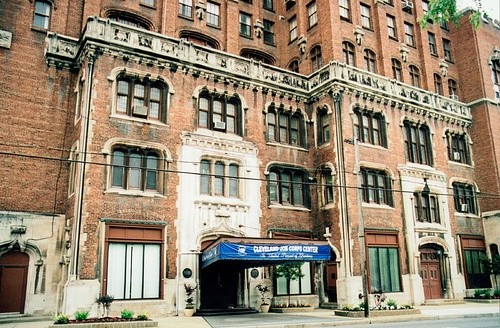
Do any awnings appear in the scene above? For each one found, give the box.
[198,237,329,268]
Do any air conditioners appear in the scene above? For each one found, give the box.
[461,204,467,212]
[133,105,148,116]
[214,121,226,129]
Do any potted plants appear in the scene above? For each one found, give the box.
[184,284,197,316]
[256,285,271,313]
